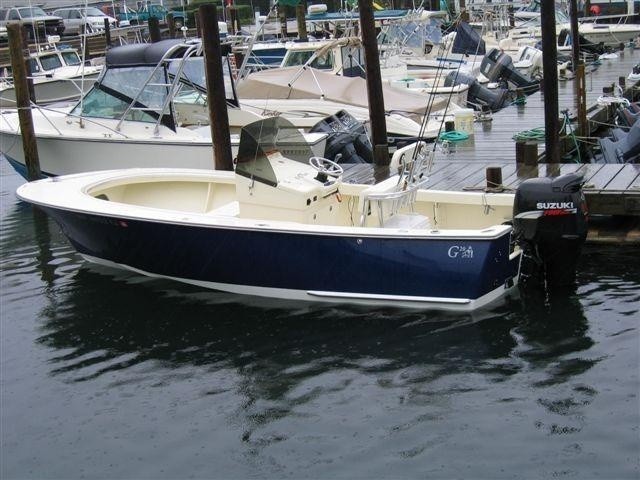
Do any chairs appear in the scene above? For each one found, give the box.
[356,139,427,219]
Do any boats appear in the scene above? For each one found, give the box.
[26,277,585,374]
[13,116,595,325]
[0,0,640,184]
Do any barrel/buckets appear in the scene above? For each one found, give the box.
[455,108,474,134]
